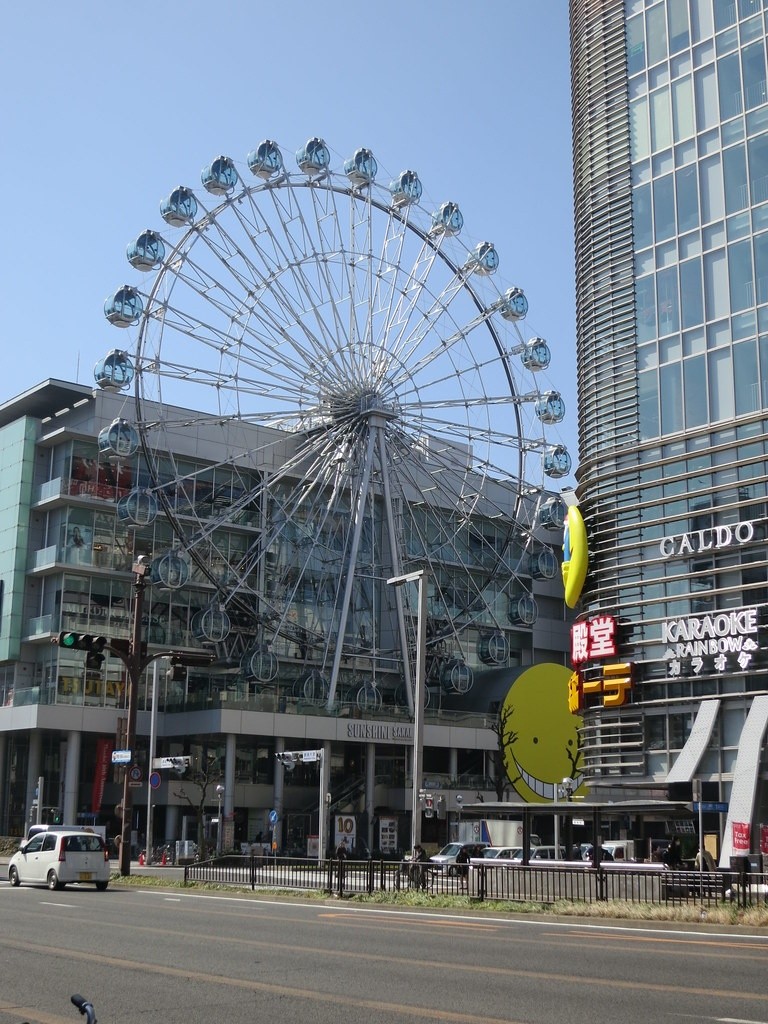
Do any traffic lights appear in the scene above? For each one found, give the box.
[58,631,106,653]
[169,649,211,668]
[165,667,187,680]
[275,752,293,763]
[424,798,434,818]
[437,800,447,820]
[83,651,106,669]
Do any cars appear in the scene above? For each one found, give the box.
[481,839,635,867]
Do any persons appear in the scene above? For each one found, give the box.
[36,836,53,850]
[472,845,484,858]
[585,841,614,860]
[412,845,426,888]
[695,846,717,895]
[256,831,263,842]
[652,835,683,870]
[337,841,347,877]
[68,837,79,850]
[456,848,470,889]
[573,843,582,860]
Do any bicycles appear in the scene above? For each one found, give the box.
[392,859,435,889]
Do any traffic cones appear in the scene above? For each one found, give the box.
[139,853,144,865]
[161,853,167,866]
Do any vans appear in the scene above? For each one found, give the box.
[27,826,85,842]
[428,842,492,877]
[8,831,111,891]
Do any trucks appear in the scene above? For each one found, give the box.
[450,819,542,848]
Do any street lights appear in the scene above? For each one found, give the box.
[386,569,432,862]
